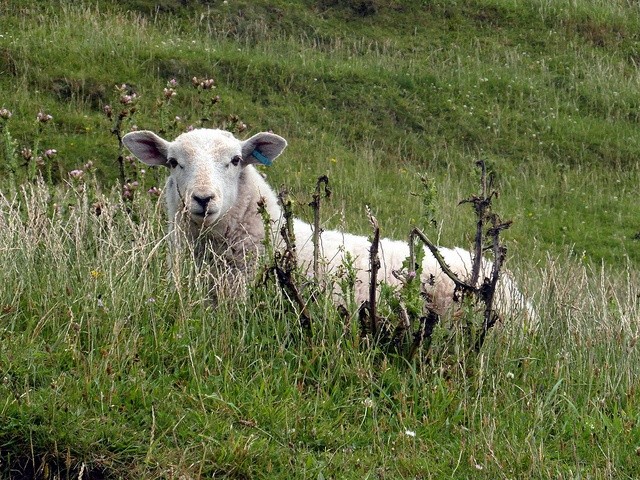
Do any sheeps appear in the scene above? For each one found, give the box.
[121,127,536,334]
